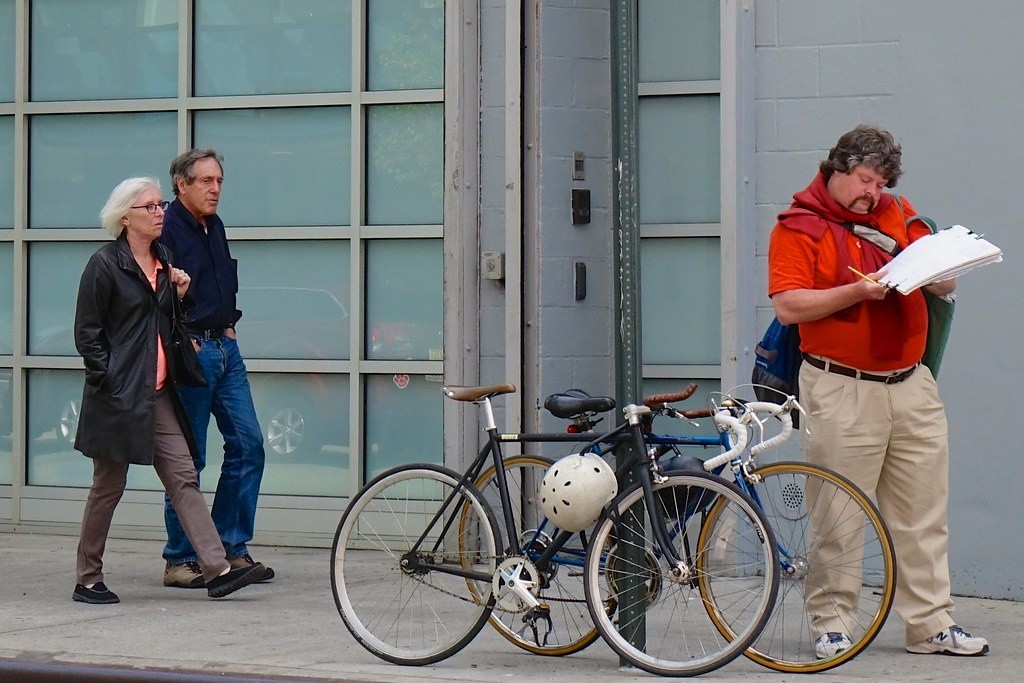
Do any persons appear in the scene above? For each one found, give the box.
[767,124,990,660]
[156,150,276,589]
[71,176,264,606]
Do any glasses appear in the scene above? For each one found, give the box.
[131,201,170,214]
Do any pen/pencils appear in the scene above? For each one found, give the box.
[848,265,892,293]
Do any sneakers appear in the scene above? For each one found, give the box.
[904,625,990,656]
[815,633,852,659]
[164,561,208,588]
[226,554,276,583]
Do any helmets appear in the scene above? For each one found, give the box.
[539,452,618,533]
[650,455,718,520]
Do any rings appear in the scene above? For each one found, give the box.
[184,273,188,276]
[181,270,184,274]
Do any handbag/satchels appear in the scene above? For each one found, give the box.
[159,243,209,389]
[752,316,804,430]
[894,194,958,382]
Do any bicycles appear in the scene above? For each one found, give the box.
[458,388,900,672]
[326,377,780,677]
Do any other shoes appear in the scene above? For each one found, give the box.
[73,581,121,604]
[206,562,266,598]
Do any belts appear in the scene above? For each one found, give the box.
[196,328,224,341]
[802,351,920,385]
